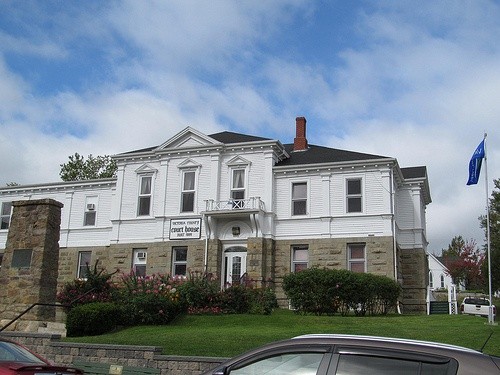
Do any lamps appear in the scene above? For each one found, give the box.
[232,227,240,235]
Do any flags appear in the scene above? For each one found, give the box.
[466,139,484,186]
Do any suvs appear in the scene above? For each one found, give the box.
[200,332,500,375]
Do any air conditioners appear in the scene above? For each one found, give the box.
[137,252,145,258]
[87,204,94,210]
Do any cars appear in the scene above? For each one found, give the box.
[0,335,85,375]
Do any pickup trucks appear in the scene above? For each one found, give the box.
[460,296,496,321]
